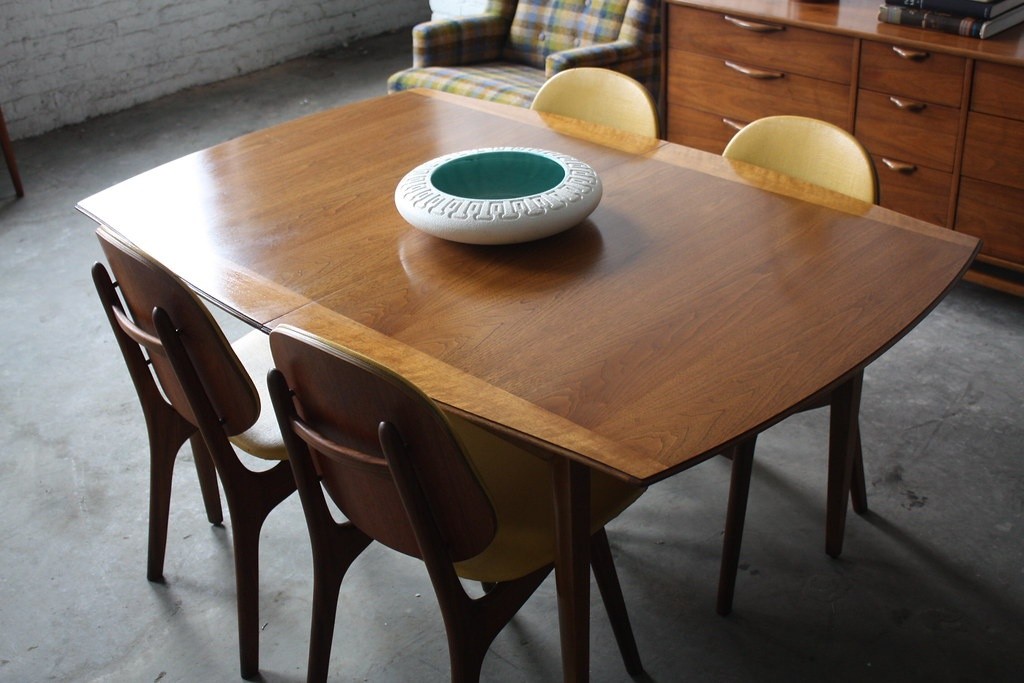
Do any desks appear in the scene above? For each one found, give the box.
[74,86,982,683]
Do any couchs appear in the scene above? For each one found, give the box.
[388,0,661,116]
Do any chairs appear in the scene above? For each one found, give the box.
[91,223,298,680]
[266,323,642,683]
[530,67,660,139]
[717,116,880,616]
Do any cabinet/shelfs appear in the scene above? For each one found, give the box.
[659,0,1024,297]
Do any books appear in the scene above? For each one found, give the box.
[886,0,1024,20]
[878,5,1024,40]
